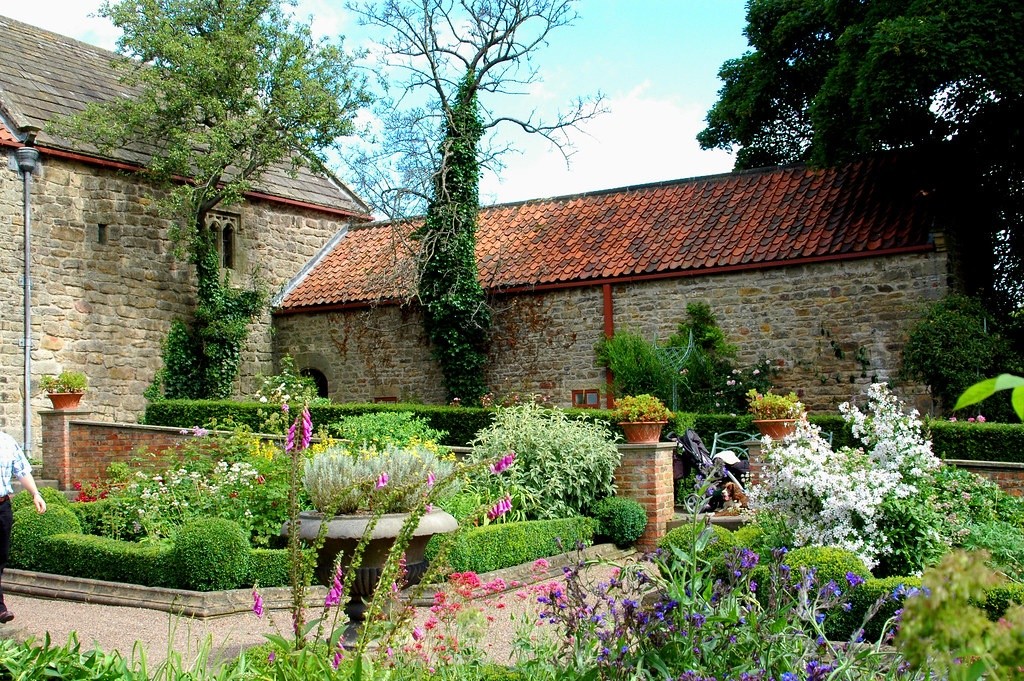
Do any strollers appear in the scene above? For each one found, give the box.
[666,428,749,513]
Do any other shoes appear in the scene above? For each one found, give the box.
[0,610,13,624]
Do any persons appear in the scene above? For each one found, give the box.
[0,430,47,624]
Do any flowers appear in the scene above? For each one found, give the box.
[611,392,676,422]
[746,385,808,420]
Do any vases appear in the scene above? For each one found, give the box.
[750,417,798,439]
[617,421,668,444]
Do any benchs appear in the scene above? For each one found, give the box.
[710,430,833,460]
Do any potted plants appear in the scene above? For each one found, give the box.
[280,439,459,659]
[38,372,88,410]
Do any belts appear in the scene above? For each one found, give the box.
[0,495,11,501]
